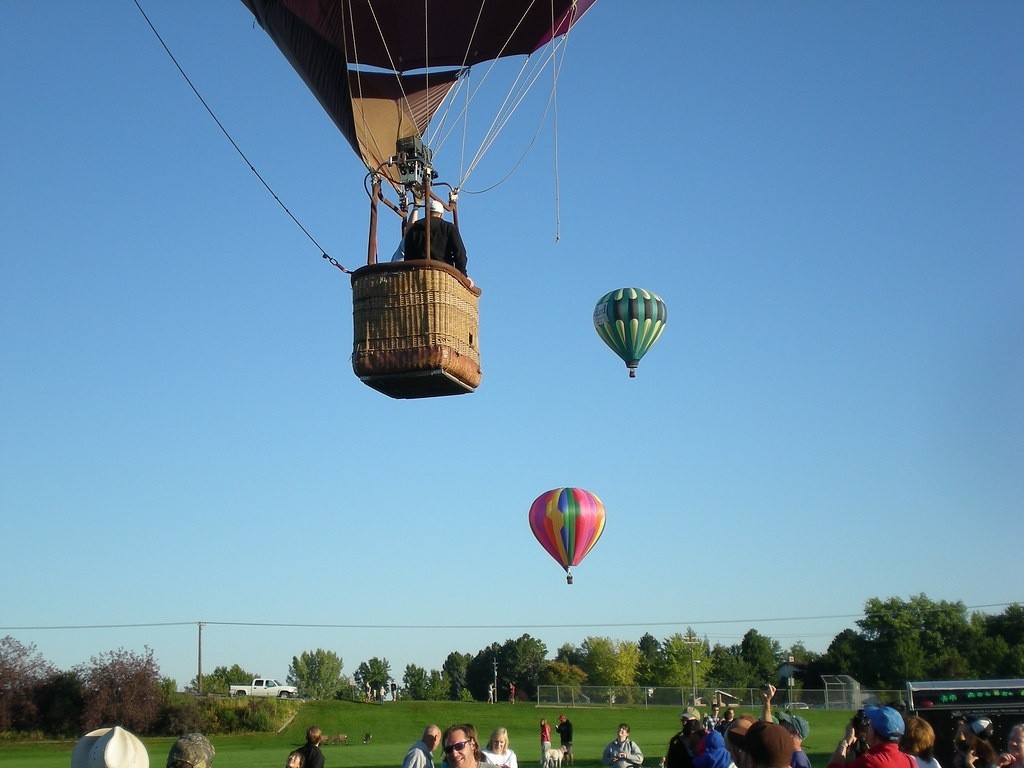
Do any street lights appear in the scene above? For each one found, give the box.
[692,660,701,706]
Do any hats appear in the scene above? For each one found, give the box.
[430,201,444,214]
[558,715,566,719]
[972,718,994,739]
[168,733,215,768]
[70,726,150,768]
[681,706,701,720]
[776,711,810,741]
[865,704,905,740]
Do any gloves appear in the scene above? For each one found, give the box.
[467,276,474,288]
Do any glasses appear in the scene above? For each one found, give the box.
[444,739,470,754]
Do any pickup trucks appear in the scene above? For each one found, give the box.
[228,678,299,698]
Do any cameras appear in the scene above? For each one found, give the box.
[853,709,870,727]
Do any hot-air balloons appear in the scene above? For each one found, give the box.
[528,487,607,584]
[593,286,668,379]
[135,1,599,399]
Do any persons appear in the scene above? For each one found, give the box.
[603,724,643,768]
[391,681,397,701]
[380,686,386,706]
[403,725,441,768]
[390,201,475,289]
[664,683,812,768]
[488,683,496,705]
[703,704,721,730]
[286,751,305,768]
[441,724,503,768]
[994,723,1024,768]
[555,715,574,766]
[540,719,551,765]
[290,726,325,768]
[507,682,515,704]
[714,708,736,737]
[166,732,215,768]
[826,701,1000,768]
[480,727,517,768]
[453,723,510,768]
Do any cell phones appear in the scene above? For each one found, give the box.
[760,684,769,691]
